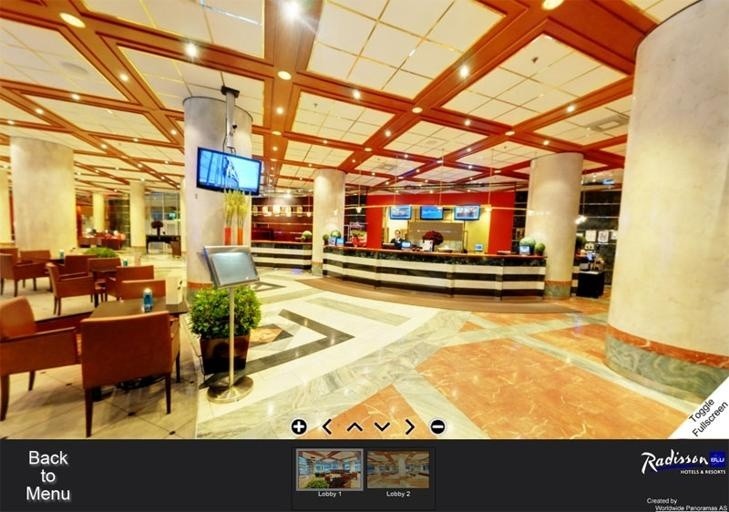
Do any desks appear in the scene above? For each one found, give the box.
[146,235,181,255]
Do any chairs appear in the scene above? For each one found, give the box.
[0,242,180,437]
[79,231,127,249]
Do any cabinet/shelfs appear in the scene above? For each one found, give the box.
[513,189,621,218]
[251,213,366,237]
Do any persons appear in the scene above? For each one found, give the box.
[391,229,404,250]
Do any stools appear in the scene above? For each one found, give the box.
[576,271,606,298]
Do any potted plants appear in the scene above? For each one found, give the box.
[535,243,545,256]
[301,231,313,242]
[323,235,329,245]
[151,221,163,237]
[222,188,247,245]
[188,287,262,374]
[331,230,341,245]
[519,237,536,255]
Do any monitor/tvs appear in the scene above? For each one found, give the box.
[519,245,530,256]
[196,146,262,196]
[454,206,480,221]
[420,205,443,220]
[402,242,411,248]
[474,242,484,252]
[388,206,412,220]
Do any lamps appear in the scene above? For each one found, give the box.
[355,148,363,213]
[306,191,312,218]
[484,135,494,212]
